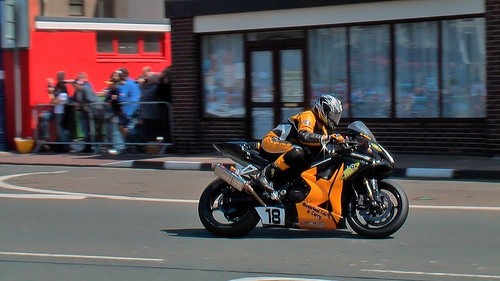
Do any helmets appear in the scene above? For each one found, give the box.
[312,94,343,130]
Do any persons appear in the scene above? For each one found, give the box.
[257,95,349,192]
[46,67,173,155]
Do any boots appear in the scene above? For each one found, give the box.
[253,161,284,192]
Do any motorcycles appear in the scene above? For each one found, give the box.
[197,121,410,239]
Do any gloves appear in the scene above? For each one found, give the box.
[321,133,345,144]
